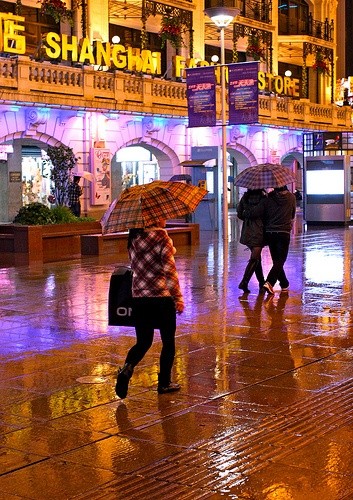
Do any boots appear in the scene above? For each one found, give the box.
[157,372,182,394]
[238,259,258,293]
[115,363,134,399]
[255,266,266,288]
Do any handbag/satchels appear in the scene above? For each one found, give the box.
[106,270,137,326]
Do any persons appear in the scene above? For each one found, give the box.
[293,188,302,200]
[236,189,269,293]
[116,220,185,398]
[68,176,83,217]
[242,186,297,294]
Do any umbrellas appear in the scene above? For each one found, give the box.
[233,162,298,191]
[99,180,209,235]
[70,170,96,184]
[169,174,193,180]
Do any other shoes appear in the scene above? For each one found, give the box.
[263,281,274,294]
[282,285,290,290]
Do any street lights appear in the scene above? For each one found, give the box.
[202,5,238,290]
